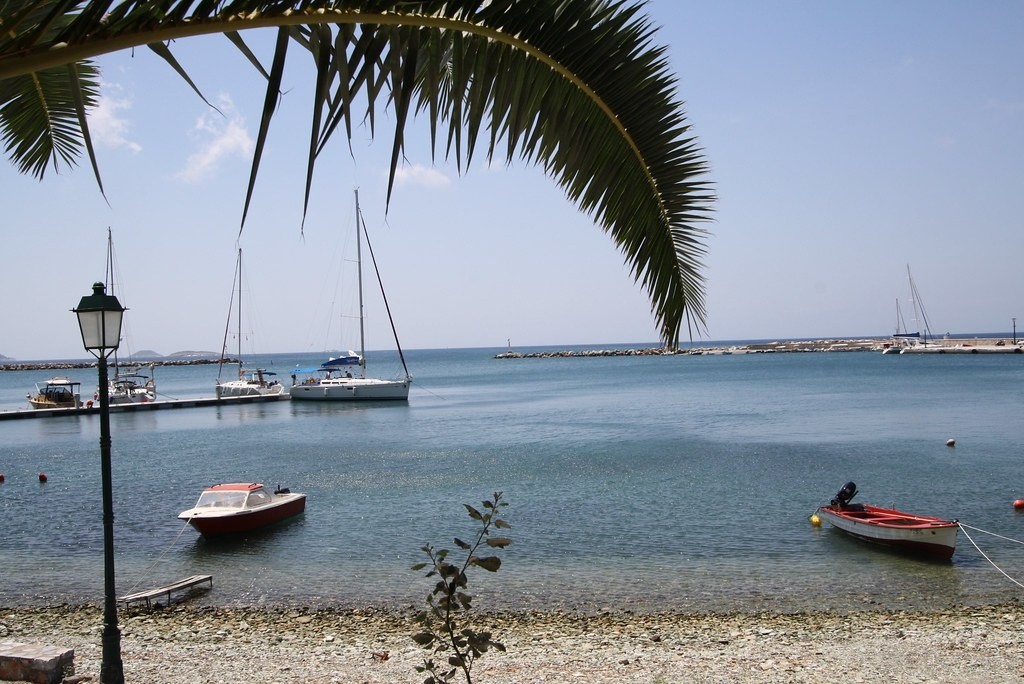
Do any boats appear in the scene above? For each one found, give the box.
[25,377,83,409]
[819,499,958,559]
[177,481,307,537]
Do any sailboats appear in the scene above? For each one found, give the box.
[213,244,284,398]
[94,225,156,403]
[882,265,1022,353]
[290,186,412,401]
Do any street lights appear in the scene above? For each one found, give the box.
[71,280,129,684]
